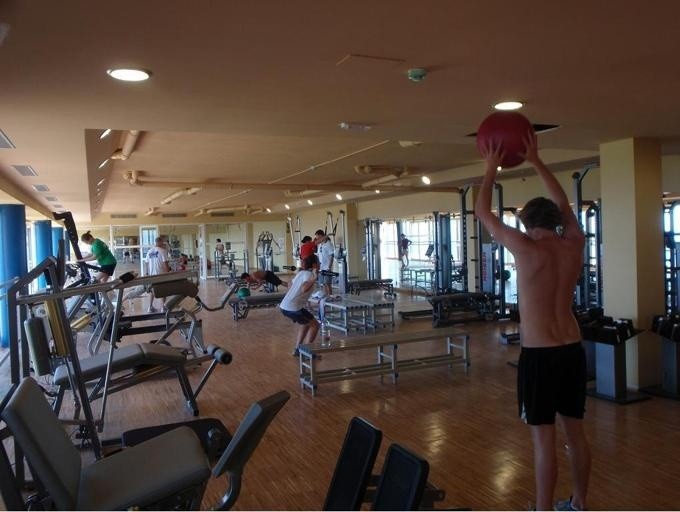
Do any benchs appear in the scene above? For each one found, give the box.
[298,295,472,397]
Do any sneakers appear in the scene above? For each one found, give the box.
[161,308,167,313]
[292,348,299,355]
[554,496,579,511]
[304,354,321,360]
[148,307,157,313]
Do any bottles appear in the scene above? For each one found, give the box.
[320,316,331,347]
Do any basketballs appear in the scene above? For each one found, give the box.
[477,111,536,168]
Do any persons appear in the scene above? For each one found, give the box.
[399,233,412,269]
[300,233,335,269]
[122,240,129,263]
[314,229,334,295]
[128,238,135,264]
[176,253,188,270]
[144,237,169,313]
[279,254,323,361]
[215,239,225,272]
[74,230,117,300]
[473,125,593,511]
[240,270,288,291]
